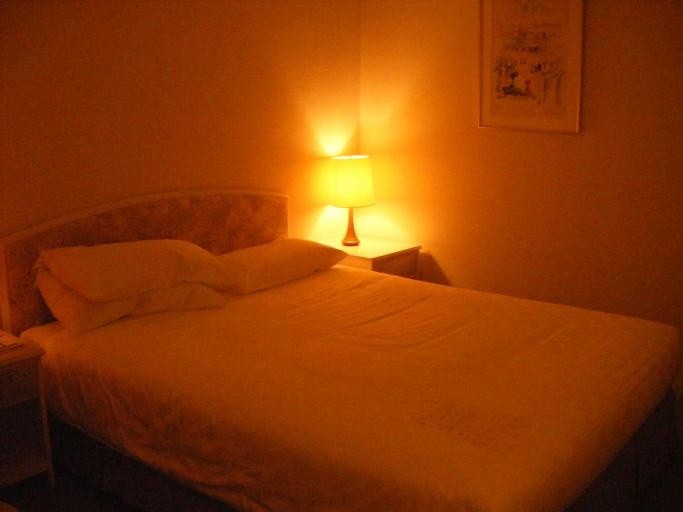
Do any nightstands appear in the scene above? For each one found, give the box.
[327,238,422,283]
[0,326,61,500]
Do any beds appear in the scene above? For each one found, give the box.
[0,186,681,512]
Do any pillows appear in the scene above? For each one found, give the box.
[219,237,346,295]
[37,232,230,297]
[35,275,227,334]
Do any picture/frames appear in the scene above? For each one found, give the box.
[470,0,588,137]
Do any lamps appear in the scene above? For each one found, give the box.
[311,156,384,250]
